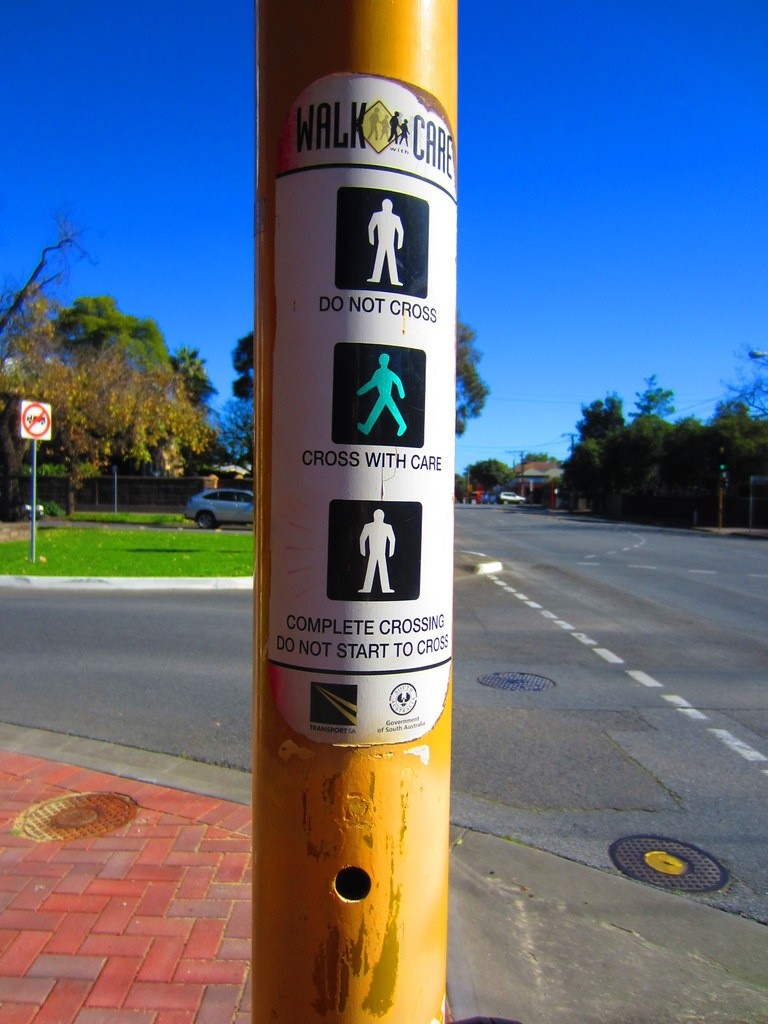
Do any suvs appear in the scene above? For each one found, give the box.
[472,490,527,505]
[184,488,254,529]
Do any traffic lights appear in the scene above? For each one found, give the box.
[719,463,729,488]
